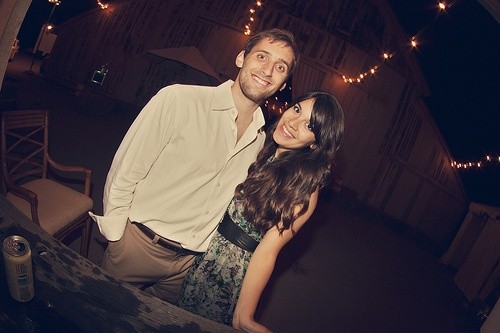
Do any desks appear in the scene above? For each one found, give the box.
[0,196,250,333]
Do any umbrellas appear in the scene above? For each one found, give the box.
[145,46,223,84]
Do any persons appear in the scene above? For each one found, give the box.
[89,28,300,305]
[176,91,345,333]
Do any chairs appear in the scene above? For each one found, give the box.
[0,110,93,260]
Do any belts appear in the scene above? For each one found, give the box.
[133,222,204,257]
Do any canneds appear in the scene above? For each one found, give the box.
[2,236,34,302]
